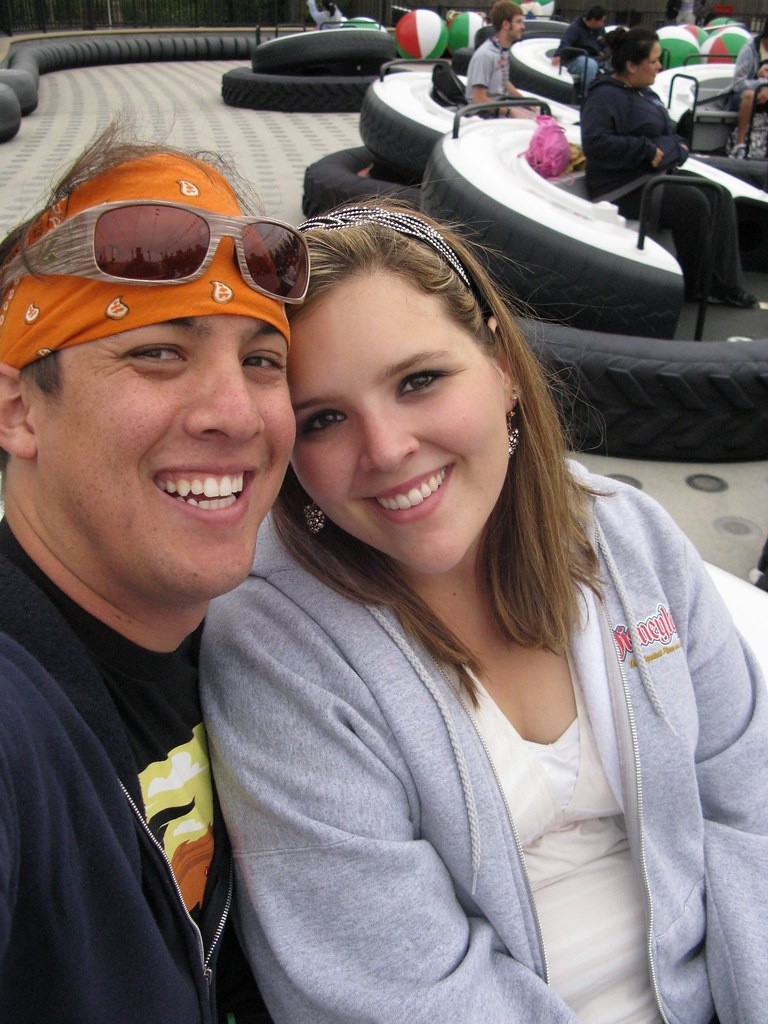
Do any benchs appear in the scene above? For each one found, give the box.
[219,14,768,462]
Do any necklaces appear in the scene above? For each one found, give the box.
[496,37,510,85]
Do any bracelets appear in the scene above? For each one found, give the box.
[505,107,511,116]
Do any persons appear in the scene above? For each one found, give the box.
[201,203,768,1024]
[306,0,341,29]
[465,1,540,118]
[553,7,610,96]
[730,21,768,158]
[0,123,311,1024]
[582,29,756,305]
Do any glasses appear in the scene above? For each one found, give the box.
[0,200,311,305]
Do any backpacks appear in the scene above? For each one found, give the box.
[432,62,468,107]
[517,113,574,187]
[726,112,768,159]
